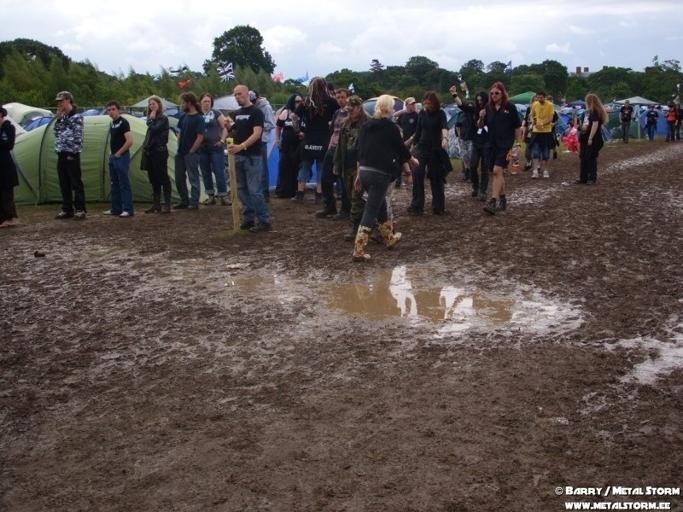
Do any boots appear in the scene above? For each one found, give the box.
[343,225,358,241]
[352,225,372,261]
[316,198,337,217]
[160,192,170,213]
[144,193,161,214]
[330,204,352,221]
[378,220,402,250]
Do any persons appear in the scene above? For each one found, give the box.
[572,93,608,185]
[351,95,421,263]
[560,92,683,154]
[0,75,560,235]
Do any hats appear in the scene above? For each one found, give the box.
[345,95,361,111]
[249,90,258,103]
[405,97,416,104]
[56,91,74,101]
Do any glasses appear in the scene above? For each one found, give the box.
[490,91,501,95]
[296,100,303,105]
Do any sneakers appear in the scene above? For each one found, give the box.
[119,211,129,218]
[543,171,550,178]
[576,178,586,185]
[313,186,322,200]
[55,210,74,219]
[249,222,272,233]
[497,198,507,212]
[511,165,521,175]
[241,221,254,230]
[531,168,538,179]
[204,197,216,205]
[483,200,497,215]
[187,203,198,210]
[222,197,232,206]
[408,205,419,211]
[433,208,443,215]
[103,209,115,215]
[587,181,594,185]
[173,203,188,209]
[291,191,304,203]
[72,210,87,220]
